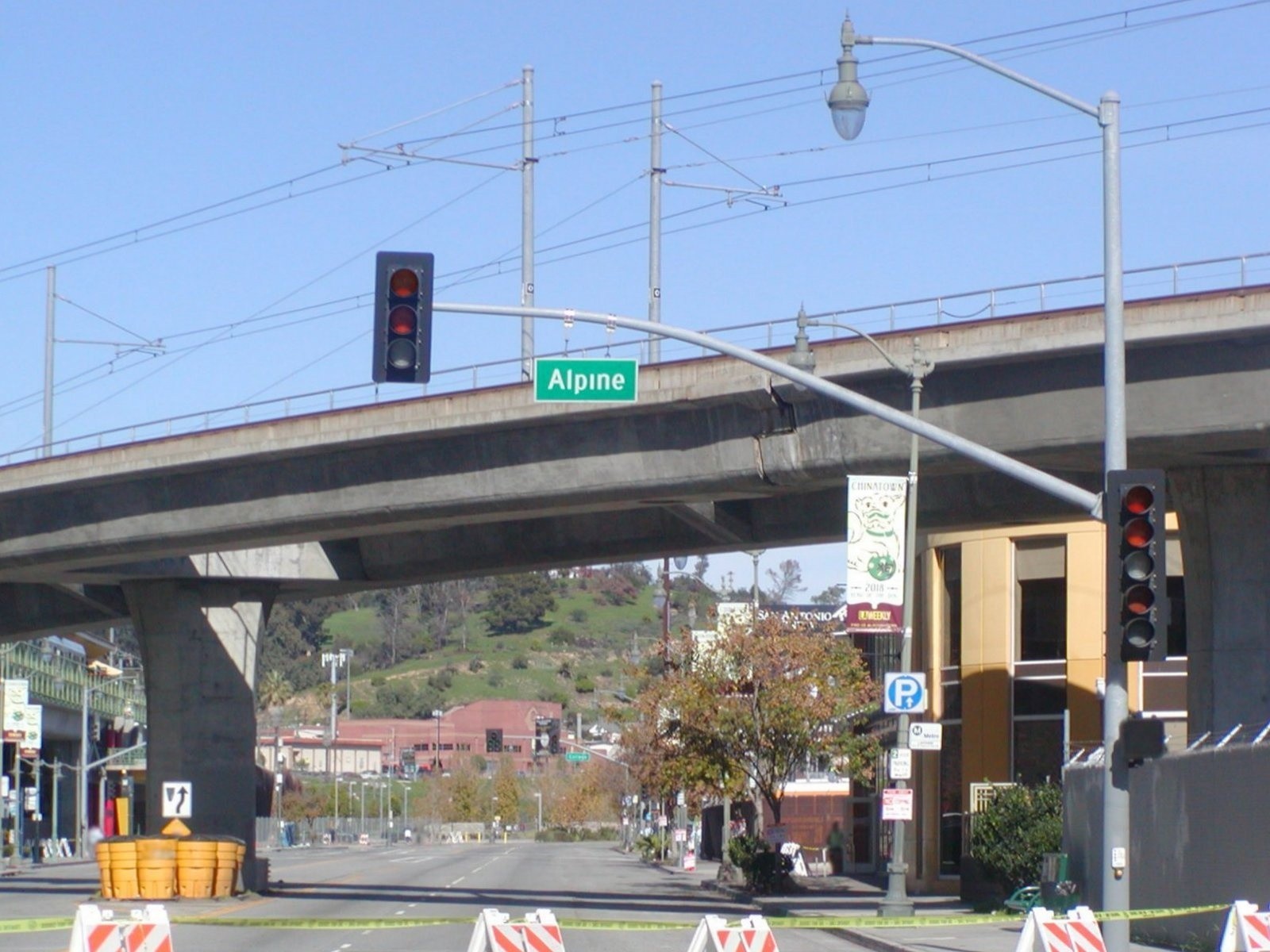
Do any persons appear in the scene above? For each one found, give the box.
[824,822,847,875]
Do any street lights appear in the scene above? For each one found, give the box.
[786,299,938,917]
[534,792,543,832]
[402,786,411,841]
[348,781,357,835]
[823,4,1133,952]
[360,781,368,833]
[378,784,387,839]
[334,777,343,843]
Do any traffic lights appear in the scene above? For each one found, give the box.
[549,732,559,753]
[486,728,503,753]
[1103,467,1168,663]
[372,248,435,384]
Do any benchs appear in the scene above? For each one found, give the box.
[1003,852,1068,913]
